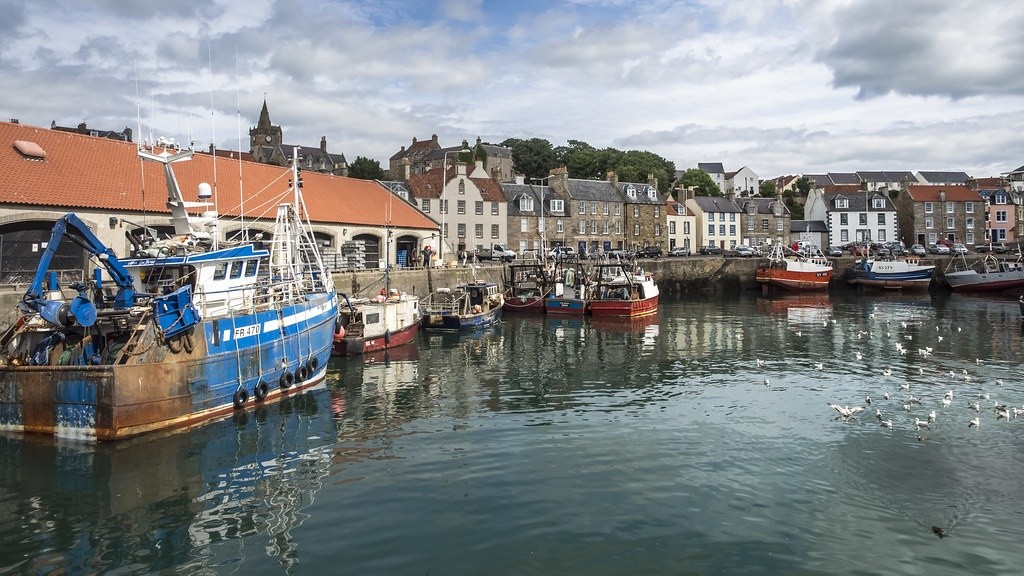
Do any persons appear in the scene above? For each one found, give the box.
[461,249,468,267]
[421,246,436,268]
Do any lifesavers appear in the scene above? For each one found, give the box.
[254,404,269,420]
[255,381,269,399]
[234,389,249,407]
[307,356,318,372]
[235,411,249,428]
[296,366,309,382]
[281,371,294,388]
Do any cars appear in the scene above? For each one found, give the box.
[728,239,970,257]
[700,245,722,255]
[667,247,691,257]
[579,248,606,260]
[607,248,630,259]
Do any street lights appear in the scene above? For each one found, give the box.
[674,185,699,260]
[439,147,472,260]
[856,189,870,259]
[529,174,556,261]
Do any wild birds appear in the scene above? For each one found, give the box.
[969,417,979,424]
[1012,407,1024,418]
[757,359,765,364]
[812,306,1004,410]
[918,435,926,441]
[764,378,770,385]
[914,418,928,426]
[929,411,936,420]
[881,419,892,426]
[876,409,882,419]
[993,401,1006,409]
[827,402,865,417]
[795,331,802,337]
[995,409,1009,419]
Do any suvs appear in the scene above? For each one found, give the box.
[548,246,575,260]
[635,246,663,259]
[974,241,1010,254]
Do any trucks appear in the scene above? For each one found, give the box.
[475,240,516,263]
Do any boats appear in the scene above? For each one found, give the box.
[941,236,1024,292]
[844,245,937,291]
[0,45,339,443]
[754,222,834,291]
[500,251,660,319]
[323,183,425,358]
[419,234,506,331]
